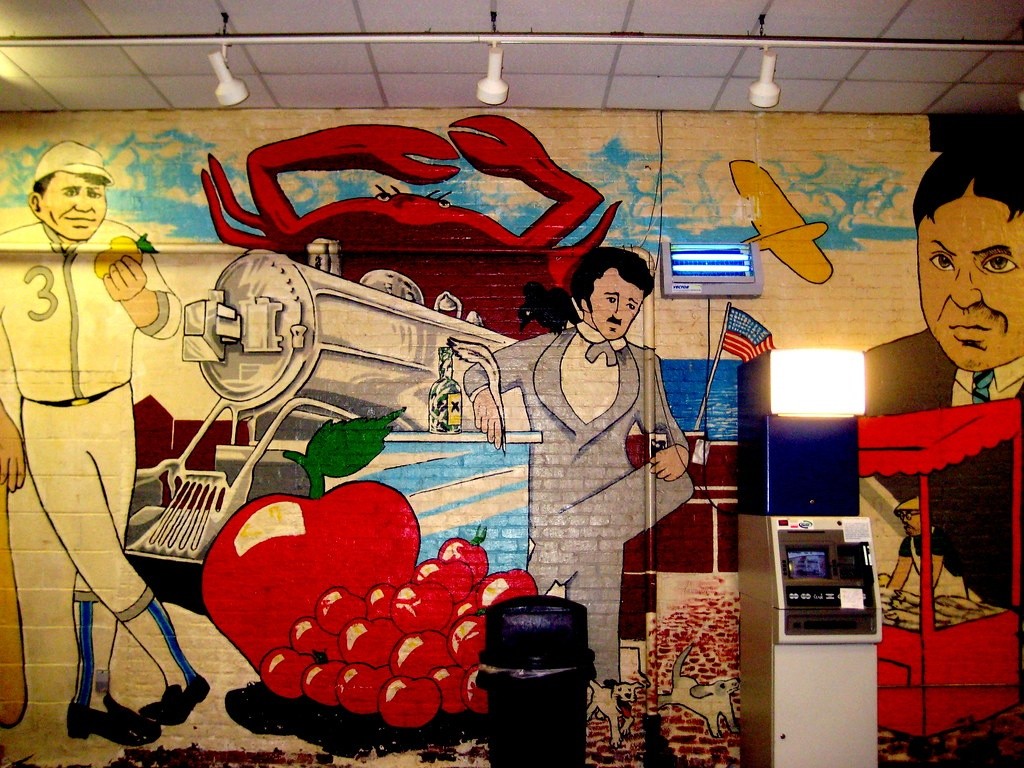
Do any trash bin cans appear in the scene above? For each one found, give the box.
[476,593,597,768]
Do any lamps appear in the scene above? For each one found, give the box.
[477,44,509,105]
[749,46,779,108]
[207,45,249,107]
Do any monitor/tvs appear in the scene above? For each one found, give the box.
[787,548,829,579]
